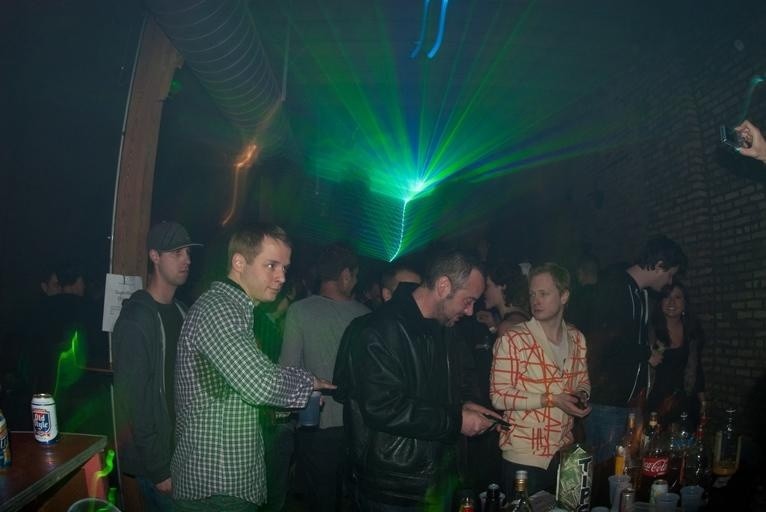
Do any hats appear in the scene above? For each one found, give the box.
[147,221,205,252]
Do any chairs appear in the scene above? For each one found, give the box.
[68,495,120,512]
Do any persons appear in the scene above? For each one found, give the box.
[732,119,766,165]
[22,267,112,434]
[111,220,704,512]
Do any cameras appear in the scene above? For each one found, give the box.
[719,124,744,149]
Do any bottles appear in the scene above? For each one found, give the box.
[460,469,534,512]
[613,391,741,489]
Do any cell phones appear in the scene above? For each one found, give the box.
[568,394,586,410]
[484,413,511,431]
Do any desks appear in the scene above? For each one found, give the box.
[0,427,109,512]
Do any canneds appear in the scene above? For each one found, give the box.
[0,410,12,469]
[649,479,669,504]
[31,394,59,445]
[621,488,637,512]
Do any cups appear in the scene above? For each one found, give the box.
[608,473,703,512]
[299,392,321,427]
[558,442,593,511]
